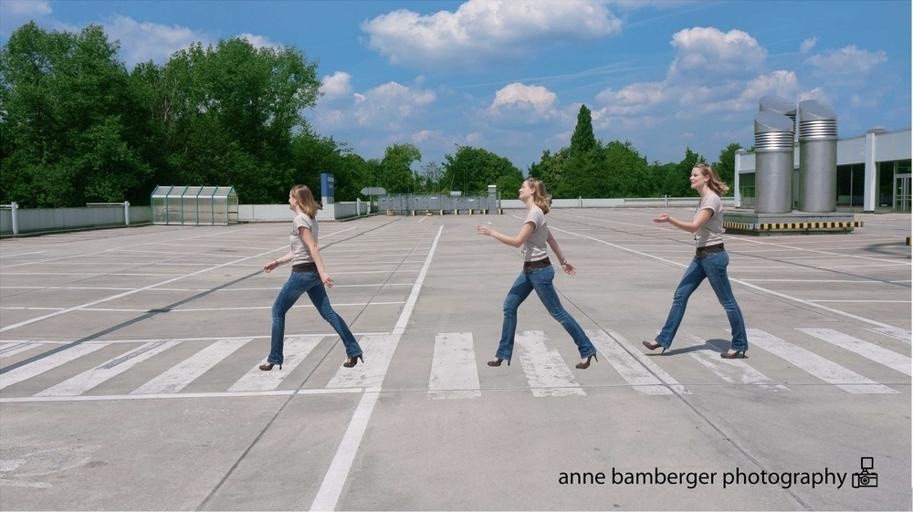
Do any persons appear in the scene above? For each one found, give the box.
[642,164,748,360]
[259,185,364,371]
[476,177,598,370]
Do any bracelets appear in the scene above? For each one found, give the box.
[274,259,279,267]
[560,259,567,265]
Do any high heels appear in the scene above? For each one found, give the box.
[721,349,745,358]
[488,358,510,366]
[576,352,598,369]
[643,341,666,354]
[259,363,281,370]
[344,353,364,367]
